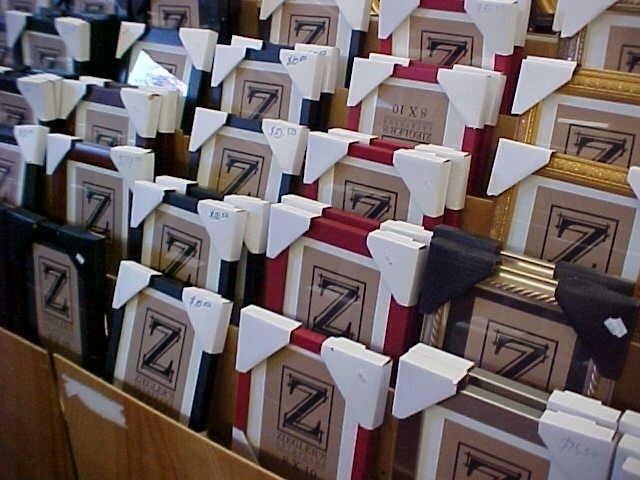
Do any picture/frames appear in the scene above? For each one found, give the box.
[1,1,640,480]
